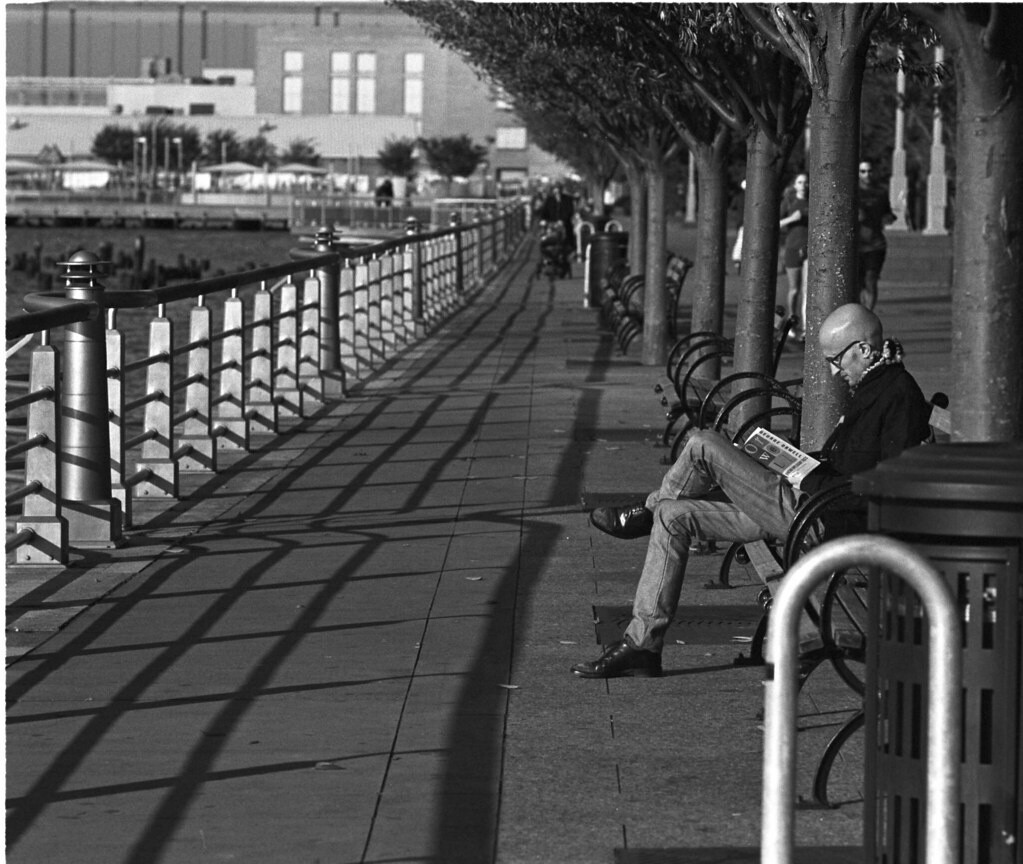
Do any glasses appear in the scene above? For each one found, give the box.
[859,169,873,174]
[825,340,861,368]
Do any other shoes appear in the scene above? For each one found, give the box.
[788,328,807,342]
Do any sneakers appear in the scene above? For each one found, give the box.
[590,501,653,539]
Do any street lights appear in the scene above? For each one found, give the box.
[137,136,147,178]
[171,137,184,178]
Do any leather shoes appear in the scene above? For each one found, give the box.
[571,639,663,678]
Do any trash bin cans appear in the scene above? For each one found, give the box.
[847,440,1023,864]
[588,231,629,307]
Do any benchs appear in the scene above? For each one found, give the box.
[602,249,949,806]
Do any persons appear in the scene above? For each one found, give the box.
[854,158,896,311]
[779,173,809,342]
[404,169,419,206]
[569,302,936,679]
[373,178,394,207]
[536,181,577,220]
